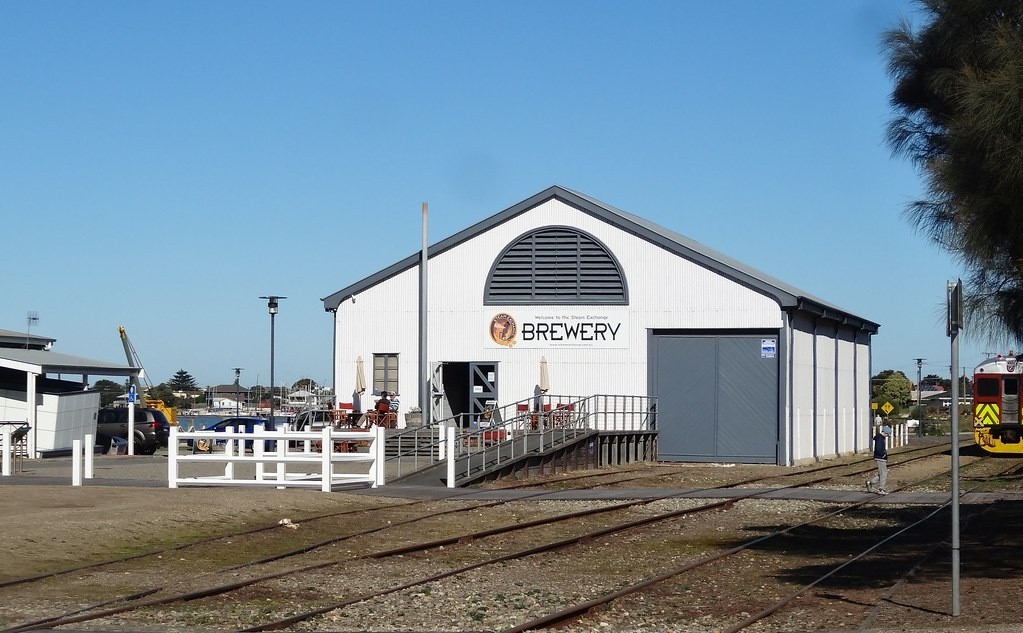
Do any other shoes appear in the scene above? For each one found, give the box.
[354,422,360,427]
[877,489,890,495]
[866,481,874,493]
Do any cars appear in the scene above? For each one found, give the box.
[187,416,277,452]
[296,410,335,443]
[97,406,171,456]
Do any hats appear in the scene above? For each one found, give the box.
[882,426,892,437]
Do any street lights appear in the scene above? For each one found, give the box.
[231,367,247,418]
[913,358,928,438]
[259,295,289,451]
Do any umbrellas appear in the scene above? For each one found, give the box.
[356,356,366,413]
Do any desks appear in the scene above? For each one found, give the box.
[348,413,363,429]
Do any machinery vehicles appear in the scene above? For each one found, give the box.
[118,325,179,425]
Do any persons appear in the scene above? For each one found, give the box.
[352,390,400,429]
[866,427,891,495]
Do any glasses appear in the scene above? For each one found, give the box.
[390,395,394,396]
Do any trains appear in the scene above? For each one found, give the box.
[972,350,1023,455]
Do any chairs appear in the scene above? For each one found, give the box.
[333,402,397,431]
[518,404,574,430]
